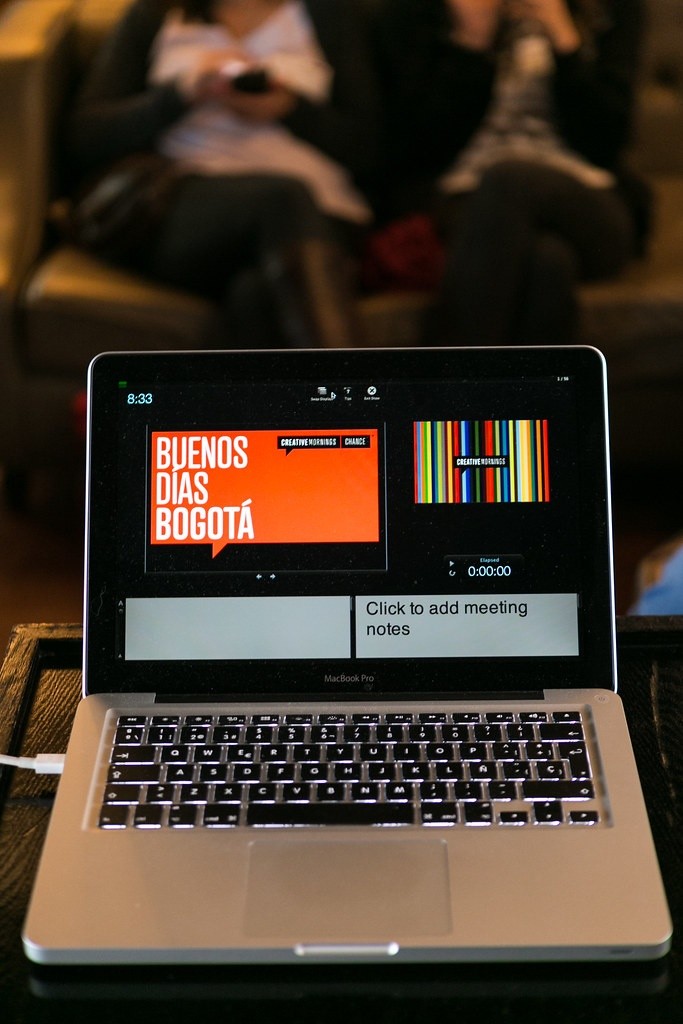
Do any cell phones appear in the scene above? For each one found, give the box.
[234,70,267,94]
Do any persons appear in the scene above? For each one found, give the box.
[57,1,658,352]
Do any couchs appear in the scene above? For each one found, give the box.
[0,1,683,514]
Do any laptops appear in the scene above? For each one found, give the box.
[23,346,673,966]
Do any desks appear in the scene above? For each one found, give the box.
[0,613,683,1024]
